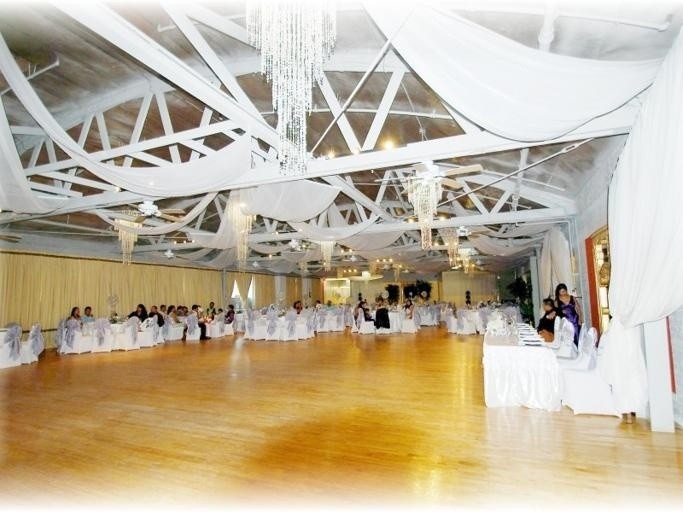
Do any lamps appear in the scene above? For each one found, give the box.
[435,225,481,279]
[243,3,338,177]
[318,238,337,274]
[402,161,443,254]
[112,209,142,267]
[224,194,257,274]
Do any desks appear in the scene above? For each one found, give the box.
[482,322,561,410]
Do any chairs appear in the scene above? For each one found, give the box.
[547,316,626,417]
[166,306,318,340]
[318,303,521,335]
[0,322,45,369]
[55,311,166,354]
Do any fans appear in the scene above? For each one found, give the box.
[373,161,483,197]
[127,200,186,224]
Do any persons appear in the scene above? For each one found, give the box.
[128,302,235,340]
[293,300,302,314]
[546,284,584,349]
[537,299,557,341]
[68,307,80,320]
[81,307,93,322]
[354,297,413,325]
[316,300,322,310]
